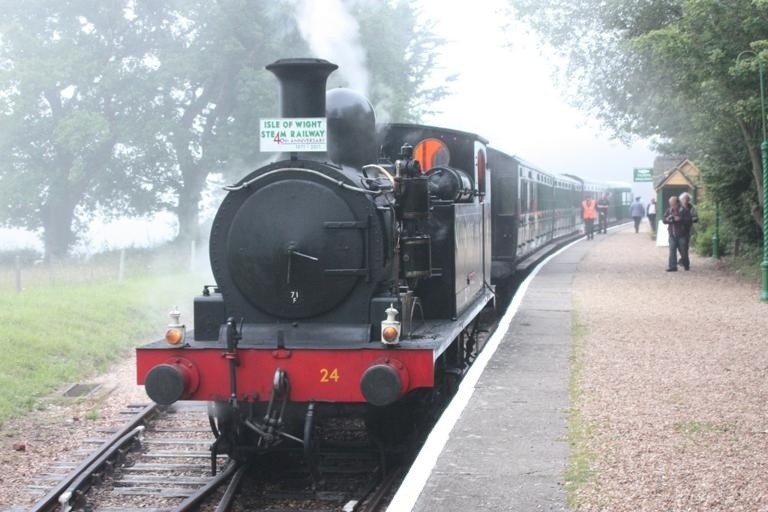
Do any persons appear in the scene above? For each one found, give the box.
[647,197,657,231]
[628,197,645,234]
[662,195,693,272]
[597,193,610,234]
[580,194,598,241]
[677,192,700,266]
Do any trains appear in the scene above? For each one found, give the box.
[135,58,633,476]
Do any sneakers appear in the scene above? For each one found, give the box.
[666,266,689,272]
